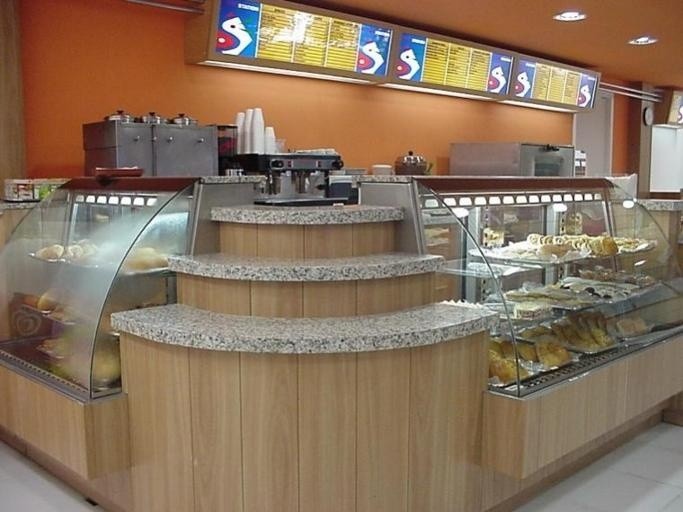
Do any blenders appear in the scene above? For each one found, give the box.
[216,124,244,175]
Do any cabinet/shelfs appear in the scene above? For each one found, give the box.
[409,174,682,397]
[0,176,199,401]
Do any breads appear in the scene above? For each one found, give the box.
[23,239,169,350]
[488,233,655,383]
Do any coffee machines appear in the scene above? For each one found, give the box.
[247,155,342,204]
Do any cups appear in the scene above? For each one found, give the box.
[297,149,336,155]
[371,164,392,175]
[234,107,277,154]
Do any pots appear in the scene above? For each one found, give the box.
[394,151,427,175]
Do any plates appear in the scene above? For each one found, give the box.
[90,167,144,178]
